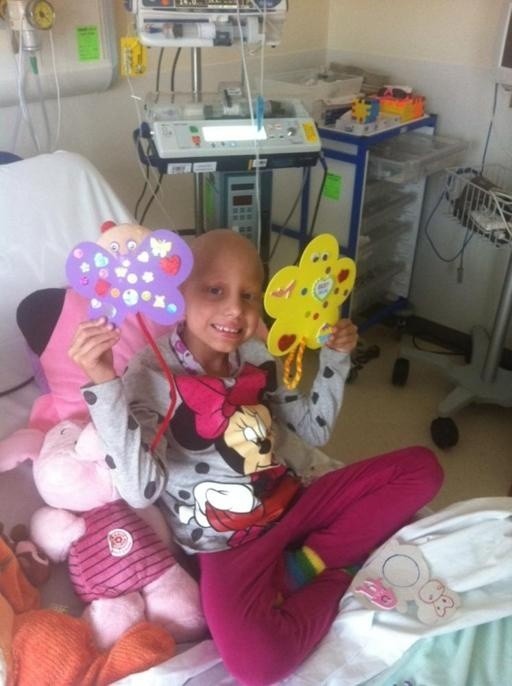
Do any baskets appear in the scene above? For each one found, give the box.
[444,162,512,249]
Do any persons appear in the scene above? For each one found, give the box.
[64,225,447,686]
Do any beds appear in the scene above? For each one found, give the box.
[0,151,510,686]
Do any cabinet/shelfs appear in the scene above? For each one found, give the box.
[271,111,437,334]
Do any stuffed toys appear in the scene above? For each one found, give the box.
[0,412,207,653]
[0,522,55,686]
[6,608,181,686]
[14,219,179,435]
